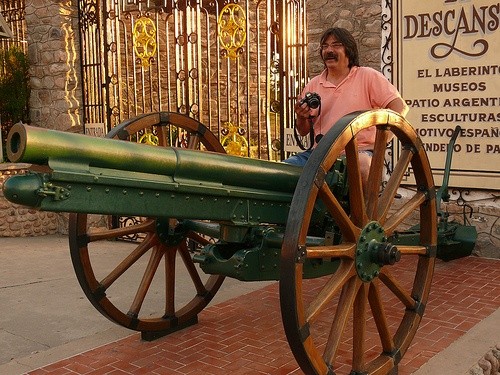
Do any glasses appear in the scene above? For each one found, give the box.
[320,42,344,50]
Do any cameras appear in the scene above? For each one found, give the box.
[299,92,321,109]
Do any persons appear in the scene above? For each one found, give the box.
[281,25,410,201]
